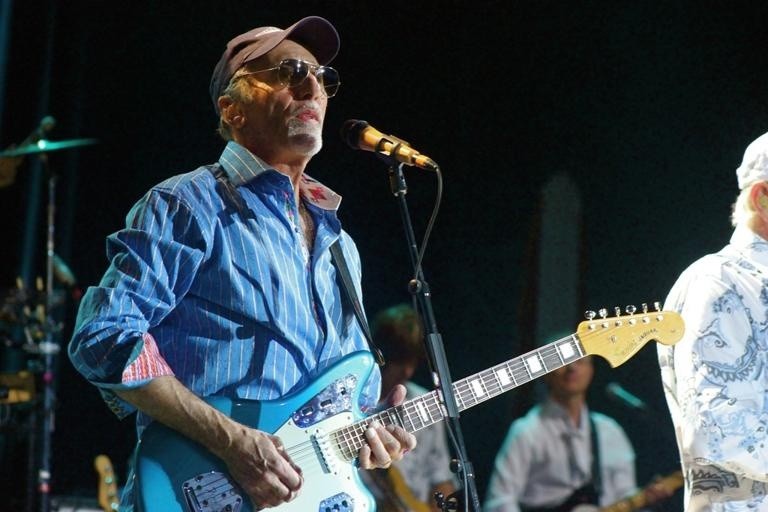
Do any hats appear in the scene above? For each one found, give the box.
[736,130,768,190]
[209,15,340,116]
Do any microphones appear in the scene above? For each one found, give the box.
[18,116,55,147]
[606,381,652,417]
[340,119,437,171]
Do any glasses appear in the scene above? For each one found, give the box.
[223,57,340,99]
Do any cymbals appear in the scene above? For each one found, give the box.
[0,138,95,157]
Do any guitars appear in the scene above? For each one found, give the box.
[131,302,683,512]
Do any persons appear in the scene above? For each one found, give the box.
[479,354,676,512]
[655,130,768,512]
[64,12,421,512]
[365,301,460,511]
